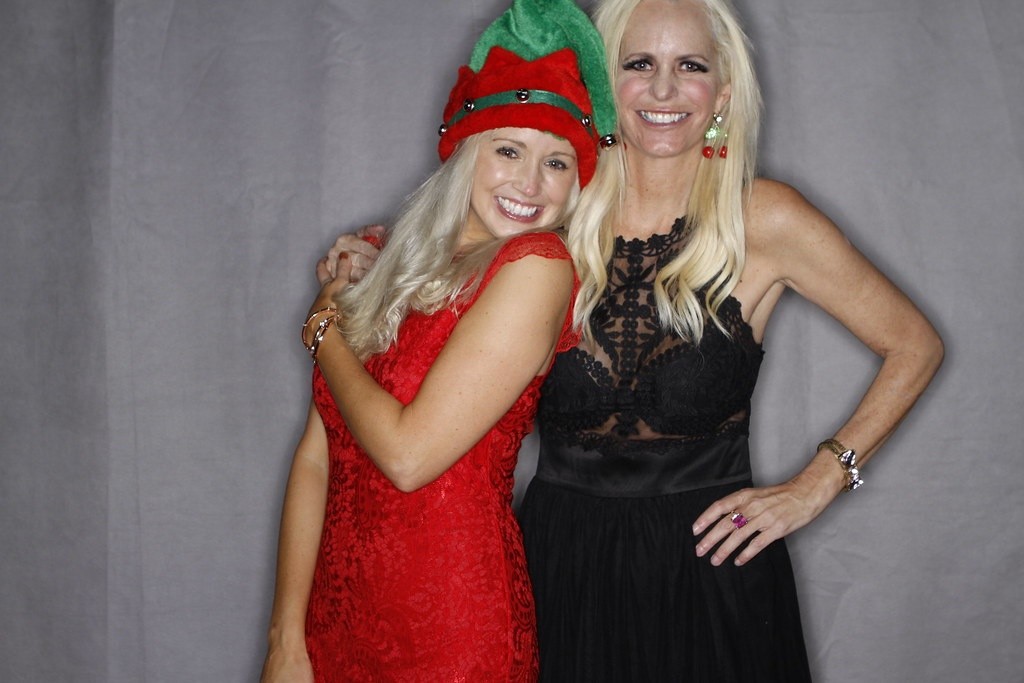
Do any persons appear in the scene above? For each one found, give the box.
[326,0,946,683]
[259,0,618,683]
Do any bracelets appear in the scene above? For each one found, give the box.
[817,439,864,493]
[302,306,340,364]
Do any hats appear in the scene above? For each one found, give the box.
[438,0,618,190]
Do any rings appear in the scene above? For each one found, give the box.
[731,511,748,530]
